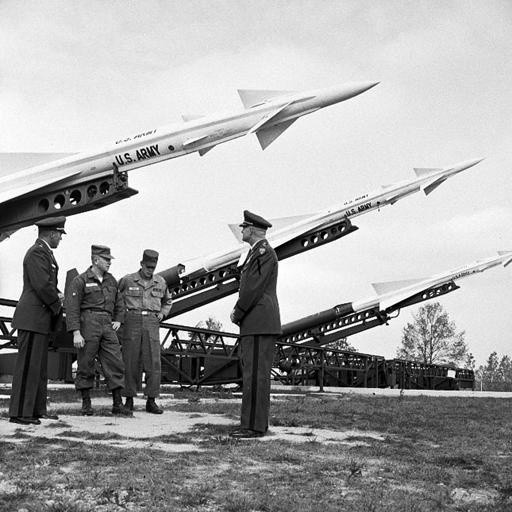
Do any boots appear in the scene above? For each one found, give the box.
[111,388,133,418]
[80,388,94,416]
[145,396,163,415]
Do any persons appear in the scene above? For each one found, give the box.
[65,244,135,416]
[10,216,68,425]
[230,210,284,438]
[116,249,174,413]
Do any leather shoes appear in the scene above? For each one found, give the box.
[36,414,60,420]
[7,416,41,426]
[228,425,269,439]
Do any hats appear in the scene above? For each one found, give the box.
[90,245,116,260]
[141,249,160,268]
[238,209,273,232]
[33,216,67,235]
